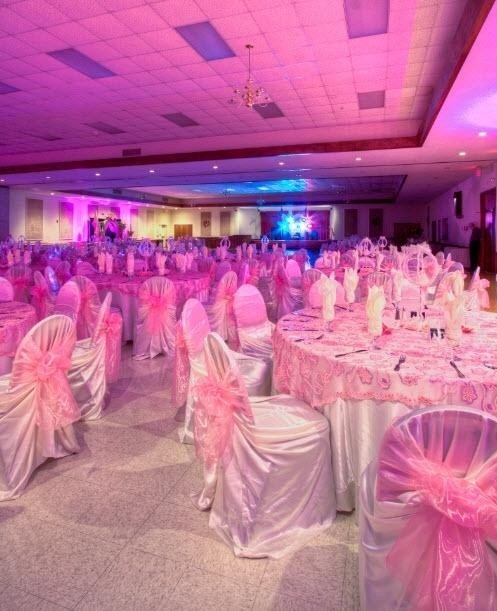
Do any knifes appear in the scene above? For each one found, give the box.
[291,312,320,318]
[450,360,466,379]
[335,349,367,358]
[335,305,354,312]
[282,329,319,332]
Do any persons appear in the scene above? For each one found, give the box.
[469,222,481,274]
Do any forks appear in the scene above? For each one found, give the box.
[295,334,325,342]
[283,318,311,322]
[393,354,405,371]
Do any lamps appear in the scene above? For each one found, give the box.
[226,45,270,112]
